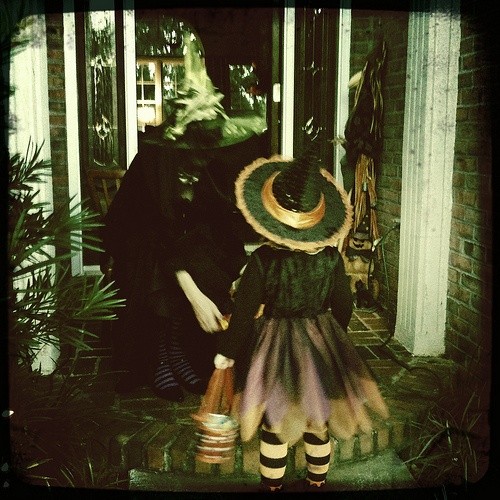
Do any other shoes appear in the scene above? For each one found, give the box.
[155,380,184,402]
[179,374,208,395]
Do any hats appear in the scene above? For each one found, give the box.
[235,141,353,251]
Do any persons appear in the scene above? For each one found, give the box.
[213,136,391,490]
[99,29,271,402]
